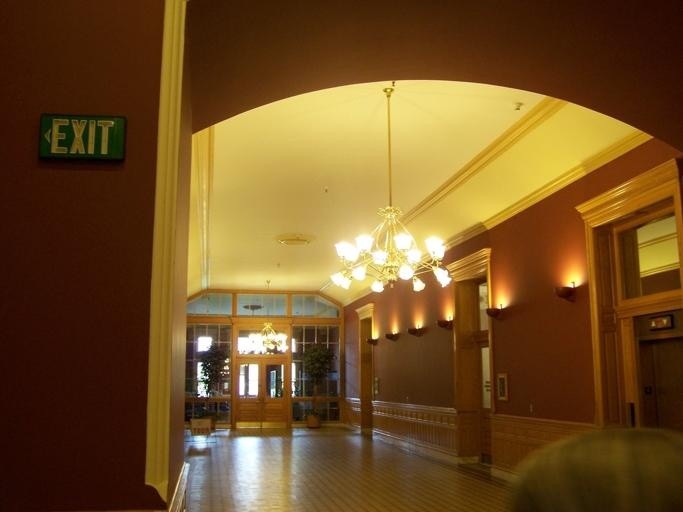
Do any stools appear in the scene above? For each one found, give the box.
[189,417,212,437]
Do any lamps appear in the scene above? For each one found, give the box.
[551,280,576,303]
[407,325,424,338]
[328,85,455,302]
[248,319,289,356]
[435,317,451,330]
[364,337,378,345]
[483,301,504,319]
[383,332,401,342]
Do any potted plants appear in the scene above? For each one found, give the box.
[199,338,227,432]
[299,338,332,428]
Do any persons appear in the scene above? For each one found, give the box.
[496,425,682,512]
